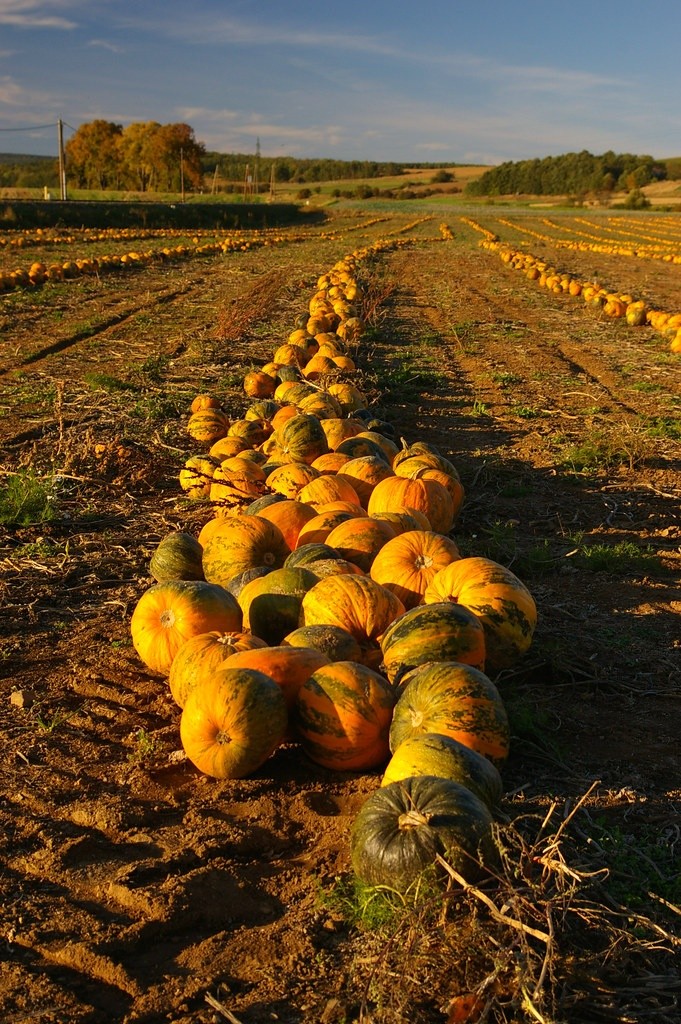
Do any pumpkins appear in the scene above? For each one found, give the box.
[440,991,489,1024]
[1,217,681,888]
